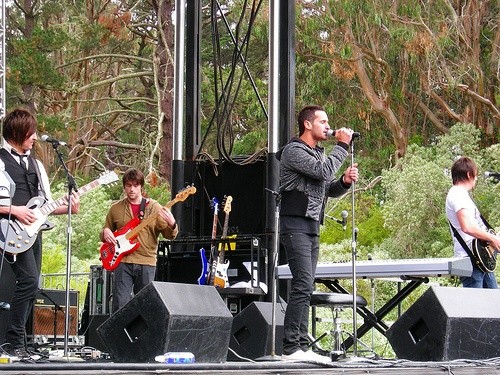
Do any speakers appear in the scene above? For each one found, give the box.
[96,281,288,365]
[384,285,500,364]
[26,290,80,335]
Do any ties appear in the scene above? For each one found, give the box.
[11,149,28,171]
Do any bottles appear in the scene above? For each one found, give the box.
[154,352,197,363]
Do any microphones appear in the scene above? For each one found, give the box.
[327,130,360,137]
[42,135,67,146]
[484,171,500,177]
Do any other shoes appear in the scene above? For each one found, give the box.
[281,349,331,364]
[1,348,49,362]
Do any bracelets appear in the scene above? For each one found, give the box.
[172,222,177,229]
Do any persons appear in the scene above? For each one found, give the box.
[446,156,500,289]
[100,169,178,308]
[0,109,79,363]
[274,105,359,362]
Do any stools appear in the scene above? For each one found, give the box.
[310,292,368,361]
